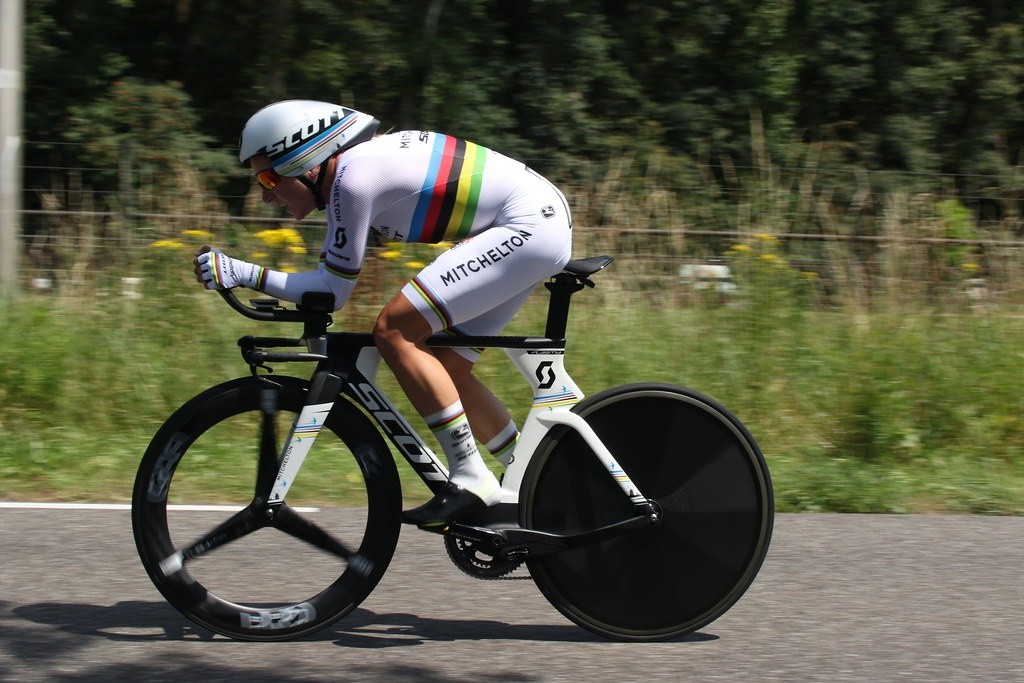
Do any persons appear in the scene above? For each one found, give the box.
[194,100,572,526]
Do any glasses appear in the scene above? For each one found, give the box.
[255,168,282,191]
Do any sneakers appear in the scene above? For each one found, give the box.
[401,471,502,525]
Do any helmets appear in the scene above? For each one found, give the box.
[238,100,381,178]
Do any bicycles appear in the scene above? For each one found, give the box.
[131,253,778,646]
